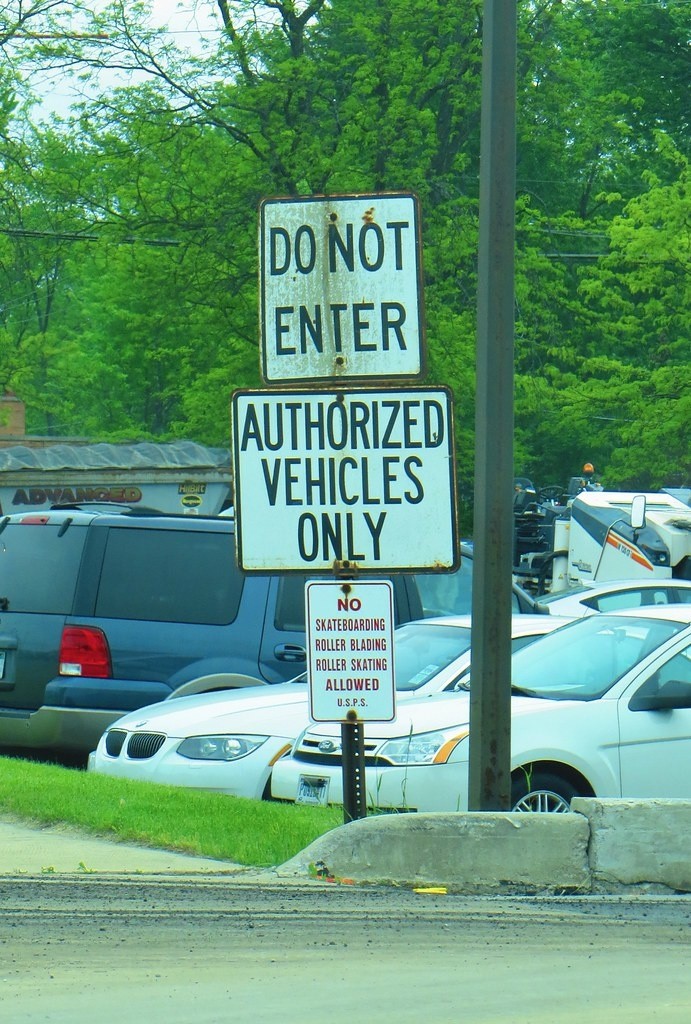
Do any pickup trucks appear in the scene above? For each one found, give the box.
[1,498,550,759]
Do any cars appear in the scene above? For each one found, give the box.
[268,596,691,818]
[86,612,691,809]
[532,576,691,619]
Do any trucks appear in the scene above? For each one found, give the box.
[512,464,691,578]
